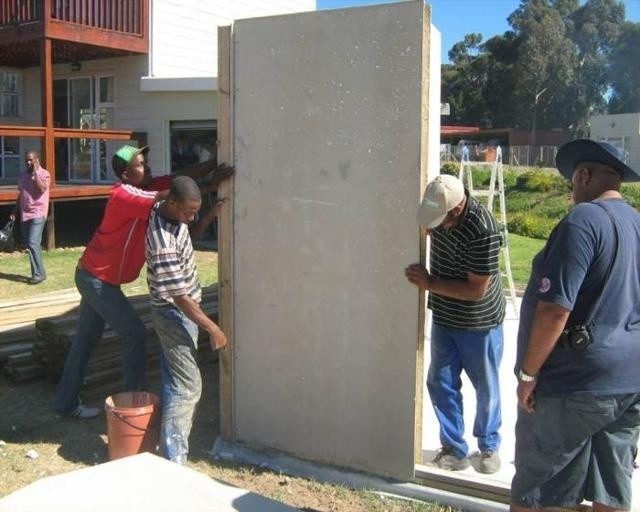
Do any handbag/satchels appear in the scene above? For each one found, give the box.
[0,220,17,252]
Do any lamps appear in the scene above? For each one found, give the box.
[70,62,81,71]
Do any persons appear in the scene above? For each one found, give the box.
[145,176,228,464]
[406,174,508,475]
[510,138,640,511]
[54,145,217,421]
[10,150,51,285]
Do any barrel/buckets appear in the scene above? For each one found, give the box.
[103,390,160,461]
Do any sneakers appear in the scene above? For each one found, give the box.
[480,449,500,473]
[57,404,100,418]
[427,446,470,471]
[30,275,46,283]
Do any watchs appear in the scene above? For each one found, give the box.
[514,365,539,383]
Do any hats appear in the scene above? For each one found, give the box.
[112,144,149,176]
[555,138,640,182]
[417,174,465,229]
